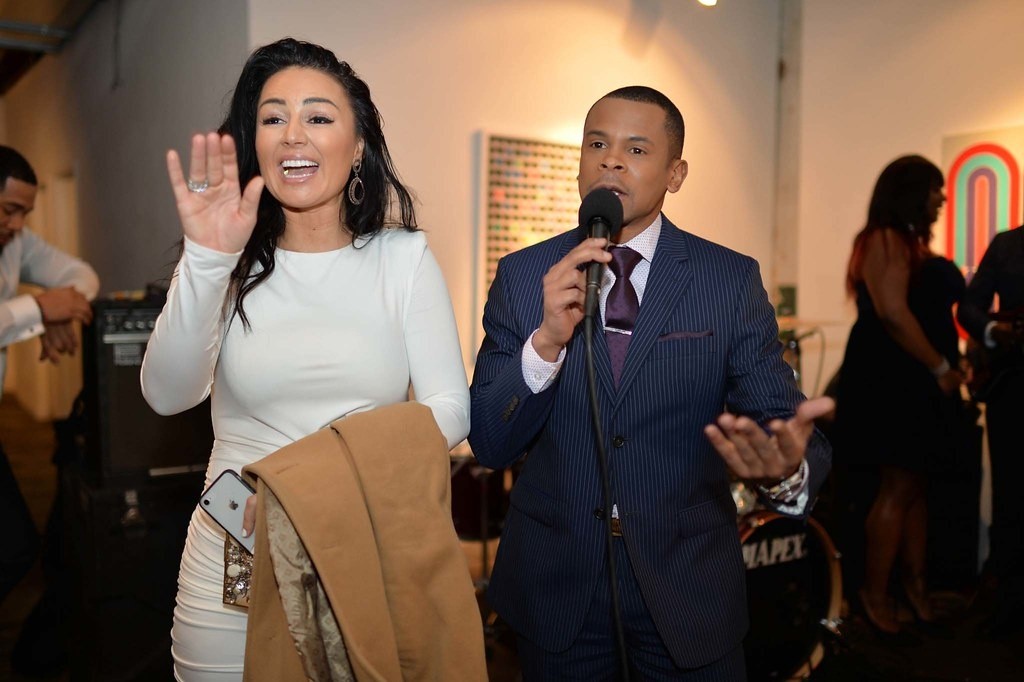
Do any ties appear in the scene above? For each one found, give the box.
[603,246,645,393]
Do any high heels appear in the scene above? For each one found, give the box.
[899,590,950,638]
[848,593,922,648]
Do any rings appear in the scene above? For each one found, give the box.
[188,179,208,194]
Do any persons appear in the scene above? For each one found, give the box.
[470,86,836,681]
[823,155,974,639]
[958,226,1022,595]
[0,144,102,598]
[141,37,471,681]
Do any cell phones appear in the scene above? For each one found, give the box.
[200,469,256,557]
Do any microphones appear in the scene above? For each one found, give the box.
[578,188,623,317]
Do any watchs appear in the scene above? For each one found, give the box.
[756,459,805,501]
